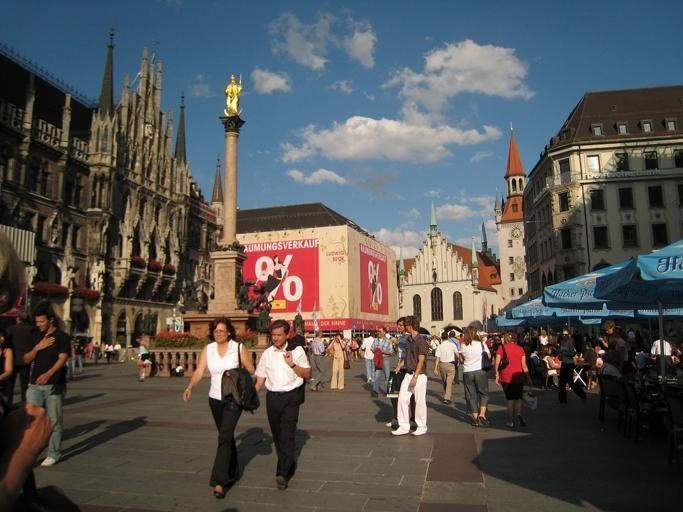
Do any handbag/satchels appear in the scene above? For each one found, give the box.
[228,343,242,411]
[481,340,493,371]
[343,349,351,369]
[498,343,509,371]
[520,373,538,412]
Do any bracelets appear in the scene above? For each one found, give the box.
[290,364,298,370]
[494,373,500,378]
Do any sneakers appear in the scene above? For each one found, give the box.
[471,418,479,427]
[506,421,514,428]
[367,377,371,386]
[410,419,416,426]
[391,427,410,436]
[479,415,488,424]
[41,457,57,467]
[320,388,326,392]
[372,390,378,397]
[442,399,450,404]
[386,417,398,428]
[275,475,287,491]
[214,485,225,499]
[311,385,316,391]
[411,427,429,436]
[372,380,375,384]
[518,412,527,427]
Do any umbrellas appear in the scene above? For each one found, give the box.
[506,294,683,348]
[593,239,683,305]
[495,306,602,342]
[542,256,683,384]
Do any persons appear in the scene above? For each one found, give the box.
[370,326,395,398]
[570,326,598,352]
[598,318,621,350]
[542,351,561,389]
[369,264,380,303]
[612,325,632,363]
[4,311,39,411]
[255,320,312,490]
[585,346,603,392]
[1,400,77,512]
[310,331,330,391]
[494,336,505,352]
[494,332,534,428]
[425,335,439,356]
[304,338,313,352]
[390,316,428,436]
[530,347,546,370]
[582,341,596,385]
[598,354,626,397]
[458,325,492,426]
[514,327,562,353]
[483,334,493,370]
[557,328,588,405]
[323,338,329,349]
[385,317,417,428]
[22,300,71,467]
[449,331,462,385]
[223,75,243,116]
[628,319,682,384]
[67,337,122,374]
[0,326,16,399]
[348,337,359,362]
[360,331,378,385]
[261,255,288,295]
[434,332,460,404]
[457,333,465,344]
[182,317,255,499]
[325,333,347,390]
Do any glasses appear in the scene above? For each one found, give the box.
[561,334,569,336]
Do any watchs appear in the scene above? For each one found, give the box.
[412,374,420,381]
